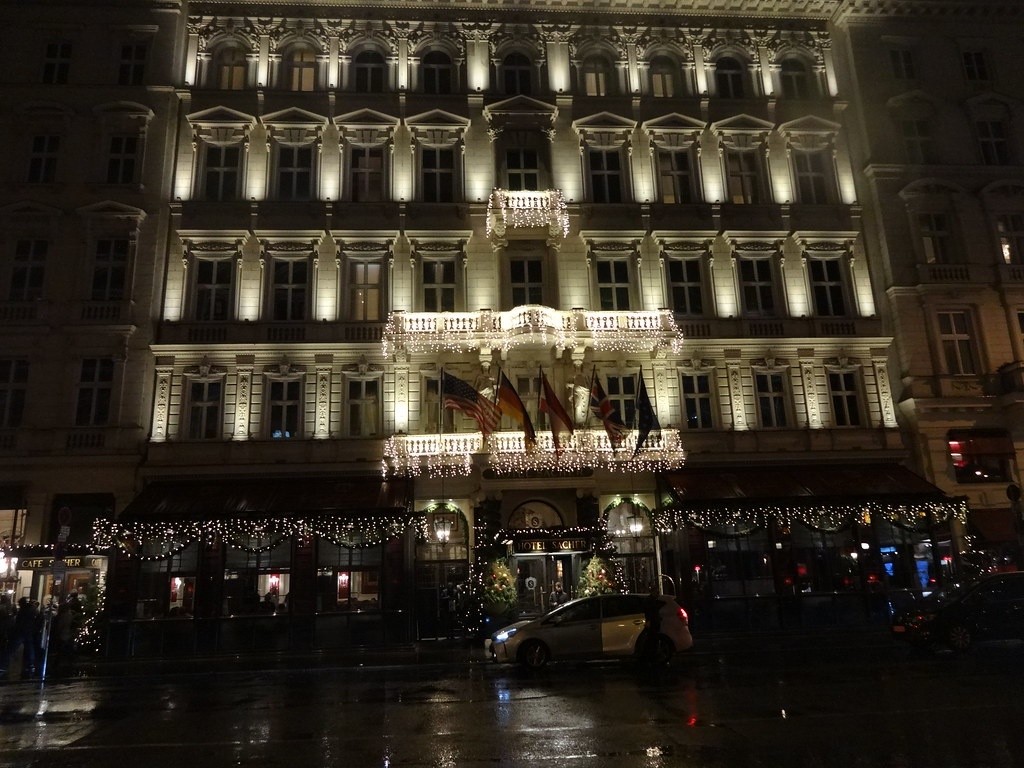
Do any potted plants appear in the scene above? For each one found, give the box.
[465,560,517,613]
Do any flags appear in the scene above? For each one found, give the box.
[498,370,537,456]
[539,368,573,461]
[443,371,502,449]
[631,370,662,460]
[589,372,630,458]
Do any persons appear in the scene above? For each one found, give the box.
[351,597,379,611]
[255,592,289,614]
[472,362,499,405]
[443,582,465,639]
[565,359,593,426]
[549,582,567,612]
[170,606,192,617]
[0,583,89,672]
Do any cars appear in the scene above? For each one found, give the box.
[489,595,692,670]
[897,571,1024,656]
